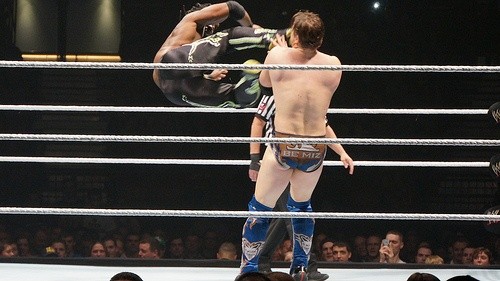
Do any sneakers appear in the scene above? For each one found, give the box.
[269,28,292,51]
[243,59,263,74]
[290,261,329,281]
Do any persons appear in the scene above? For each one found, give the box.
[249,92,353,281]
[153,2,292,109]
[240,12,342,273]
[0,215,500,281]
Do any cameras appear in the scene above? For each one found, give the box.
[382,239,390,246]
